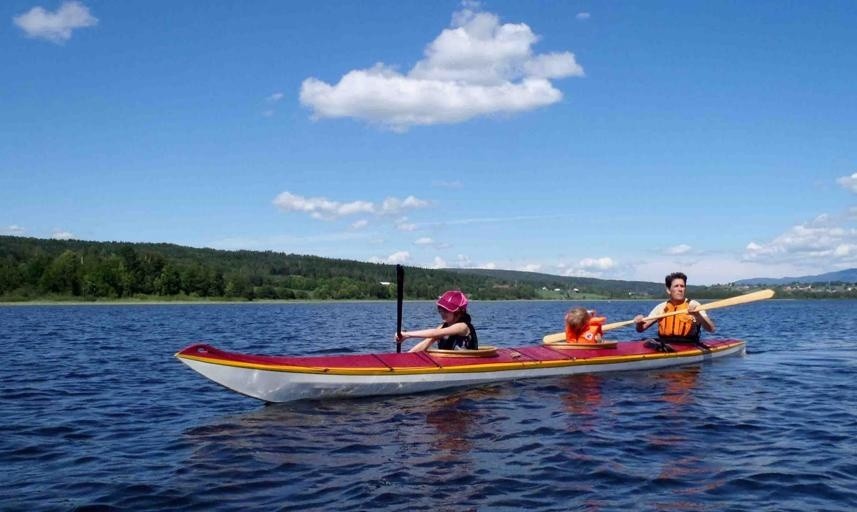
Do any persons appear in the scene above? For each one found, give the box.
[391,288,482,355]
[416,390,488,488]
[555,369,608,432]
[632,272,717,343]
[561,306,608,344]
[651,365,703,421]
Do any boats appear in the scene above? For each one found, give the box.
[173,327,748,406]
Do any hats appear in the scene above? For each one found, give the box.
[436,290,467,313]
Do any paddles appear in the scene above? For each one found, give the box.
[543,288,774,344]
[397,265,404,351]
